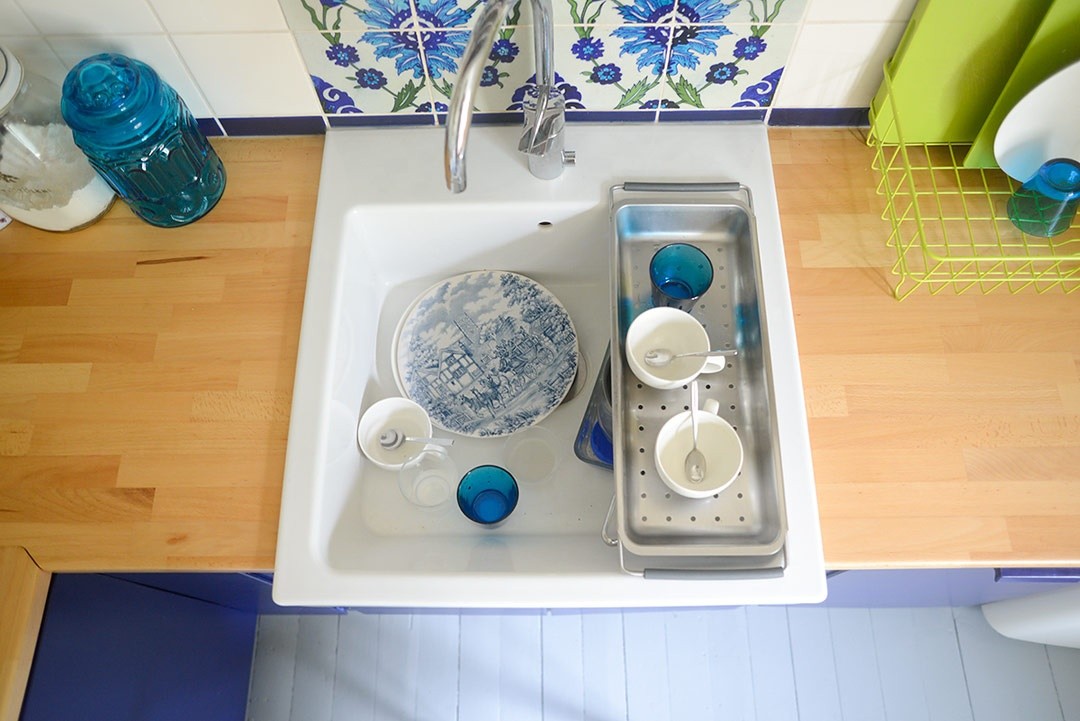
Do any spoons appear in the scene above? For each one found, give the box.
[685,378,706,485]
[380,429,457,451]
[644,345,738,369]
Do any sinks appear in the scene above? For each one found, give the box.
[270,127,828,609]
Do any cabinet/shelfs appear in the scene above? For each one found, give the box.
[785,569,1080,615]
[22,576,253,721]
[109,572,349,621]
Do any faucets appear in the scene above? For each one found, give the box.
[443,1,578,194]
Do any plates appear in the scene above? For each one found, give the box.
[389,270,579,440]
[994,61,1080,182]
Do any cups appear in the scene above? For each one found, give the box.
[649,243,714,313]
[357,396,446,472]
[395,448,457,509]
[654,395,744,500]
[507,427,559,488]
[1010,159,1080,241]
[0,45,119,235]
[457,466,519,530]
[59,52,226,228]
[626,306,727,391]
